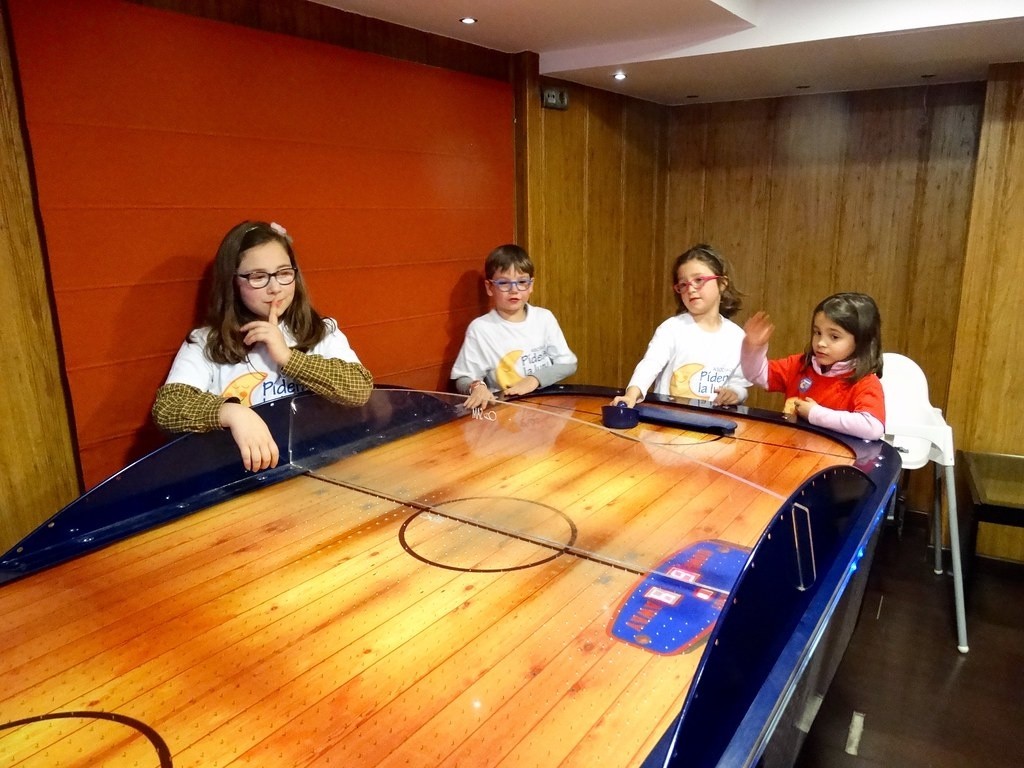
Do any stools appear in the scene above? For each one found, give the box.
[952,450,1024,586]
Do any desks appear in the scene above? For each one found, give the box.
[0,384,899,768]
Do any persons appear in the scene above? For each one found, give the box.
[151,219,373,473]
[450,244,578,410]
[741,291,886,441]
[608,243,755,410]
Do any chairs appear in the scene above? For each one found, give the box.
[878,352,969,654]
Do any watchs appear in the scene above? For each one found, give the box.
[469,380,487,395]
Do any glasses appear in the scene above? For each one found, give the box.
[673,275,720,293]
[236,267,298,289]
[489,278,533,292]
